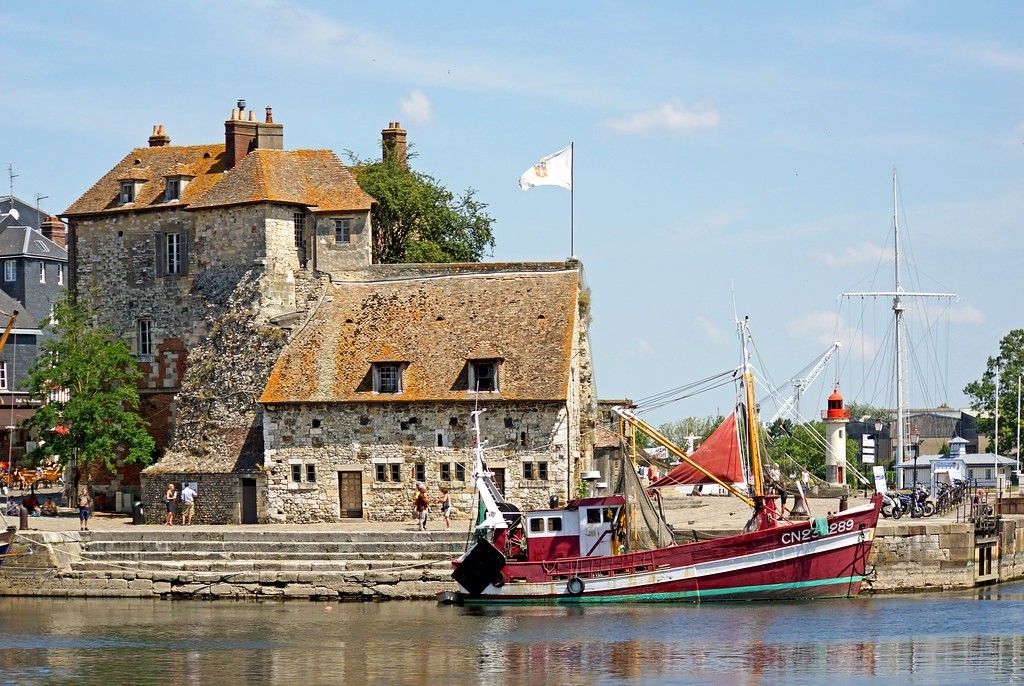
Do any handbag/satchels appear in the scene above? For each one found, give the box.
[161,493,170,503]
[412,505,419,519]
[443,500,449,509]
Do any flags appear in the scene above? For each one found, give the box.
[519,145,572,190]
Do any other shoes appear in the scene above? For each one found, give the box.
[181,523,186,526]
[81,527,83,530]
[85,527,89,530]
[443,528,450,531]
[187,523,191,526]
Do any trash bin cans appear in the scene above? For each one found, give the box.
[549,495,558,508]
[131,500,144,523]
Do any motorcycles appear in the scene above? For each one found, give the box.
[881,478,965,518]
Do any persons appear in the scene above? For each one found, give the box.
[165,484,176,526]
[769,463,811,494]
[436,488,451,532]
[0,460,63,516]
[974,489,986,515]
[182,483,197,526]
[414,481,429,533]
[78,488,91,531]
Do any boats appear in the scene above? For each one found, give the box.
[451,314,884,606]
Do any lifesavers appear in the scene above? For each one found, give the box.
[567,577,585,597]
[490,571,506,588]
